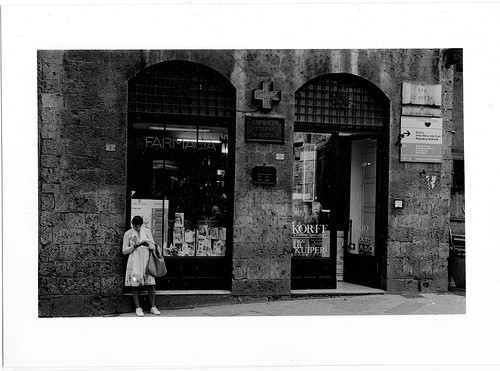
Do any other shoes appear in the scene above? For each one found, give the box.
[150,307,160,315]
[135,307,144,316]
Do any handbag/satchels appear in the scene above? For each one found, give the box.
[146,243,168,279]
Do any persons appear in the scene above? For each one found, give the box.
[121,216,161,316]
